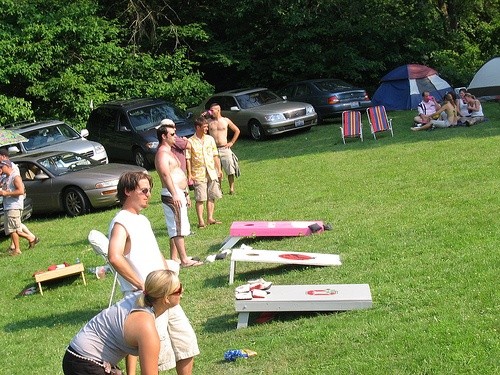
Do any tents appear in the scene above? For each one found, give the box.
[372,64,453,111]
[467,56,500,102]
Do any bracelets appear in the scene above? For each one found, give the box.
[231,141,234,144]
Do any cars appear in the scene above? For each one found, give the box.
[186,86,318,141]
[275,78,371,125]
[5,151,150,218]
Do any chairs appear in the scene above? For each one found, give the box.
[87,229,181,309]
[28,157,43,175]
[365,105,394,141]
[20,167,36,180]
[339,111,363,145]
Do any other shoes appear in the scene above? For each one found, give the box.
[6,246,15,252]
[33,234,40,244]
[27,239,35,249]
[9,250,22,257]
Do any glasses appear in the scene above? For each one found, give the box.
[168,132,175,136]
[167,282,183,296]
[135,187,151,194]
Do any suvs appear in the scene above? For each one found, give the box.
[87,98,205,171]
[0,119,108,172]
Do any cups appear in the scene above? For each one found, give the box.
[96,266,105,280]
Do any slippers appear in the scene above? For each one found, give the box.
[183,260,206,267]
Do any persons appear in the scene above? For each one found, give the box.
[106,172,201,375]
[447,92,456,105]
[62,270,184,375]
[414,91,441,127]
[185,117,223,229]
[0,160,40,256]
[457,89,472,121]
[410,95,457,131]
[459,93,484,127]
[154,124,203,268]
[0,148,35,253]
[155,118,197,236]
[201,103,240,195]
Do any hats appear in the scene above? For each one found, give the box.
[155,119,176,130]
[0,160,12,167]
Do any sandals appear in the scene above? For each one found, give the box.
[209,219,223,225]
[198,224,207,229]
